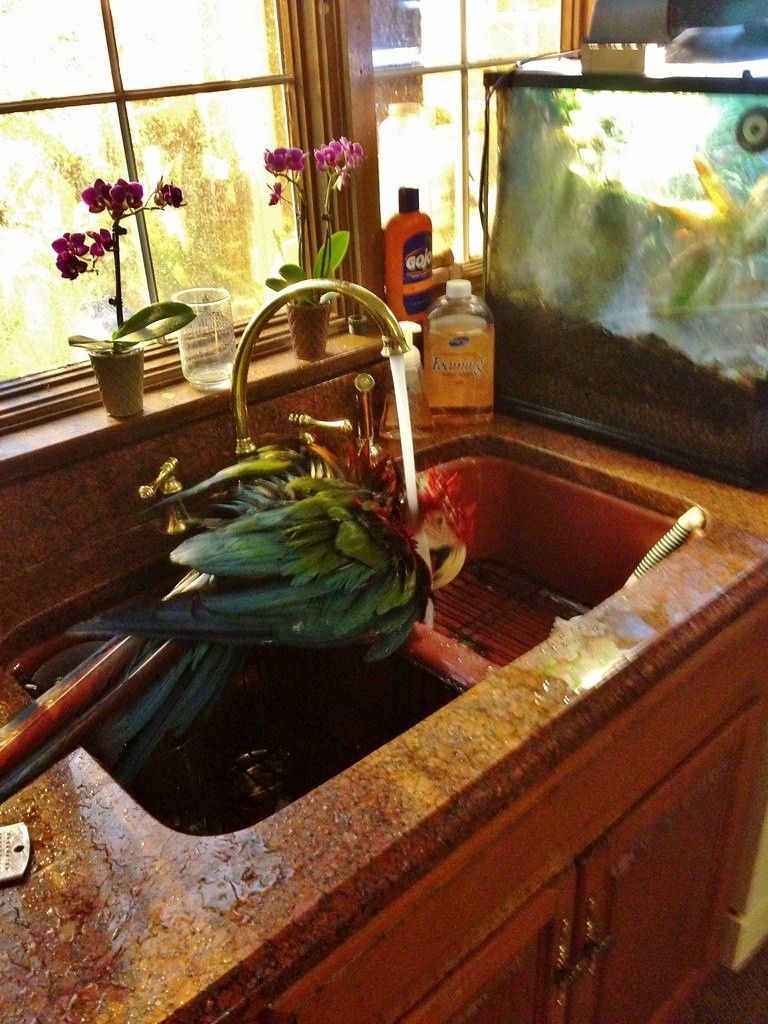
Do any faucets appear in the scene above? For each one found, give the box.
[228,275,413,492]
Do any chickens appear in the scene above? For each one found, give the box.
[0,423,474,807]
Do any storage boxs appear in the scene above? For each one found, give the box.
[476,66,768,495]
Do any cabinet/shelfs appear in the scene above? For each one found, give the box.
[266,610,768,1024]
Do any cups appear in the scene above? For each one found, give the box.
[171,287,237,391]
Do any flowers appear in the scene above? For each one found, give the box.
[54,178,193,353]
[260,138,359,303]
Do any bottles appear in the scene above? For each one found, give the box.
[382,187,436,322]
[422,280,496,426]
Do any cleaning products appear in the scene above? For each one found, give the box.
[422,278,497,427]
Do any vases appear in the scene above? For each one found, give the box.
[87,350,146,418]
[285,304,332,360]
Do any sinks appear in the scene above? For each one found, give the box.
[383,432,712,670]
[6,614,478,838]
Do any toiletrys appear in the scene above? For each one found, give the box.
[384,185,436,326]
